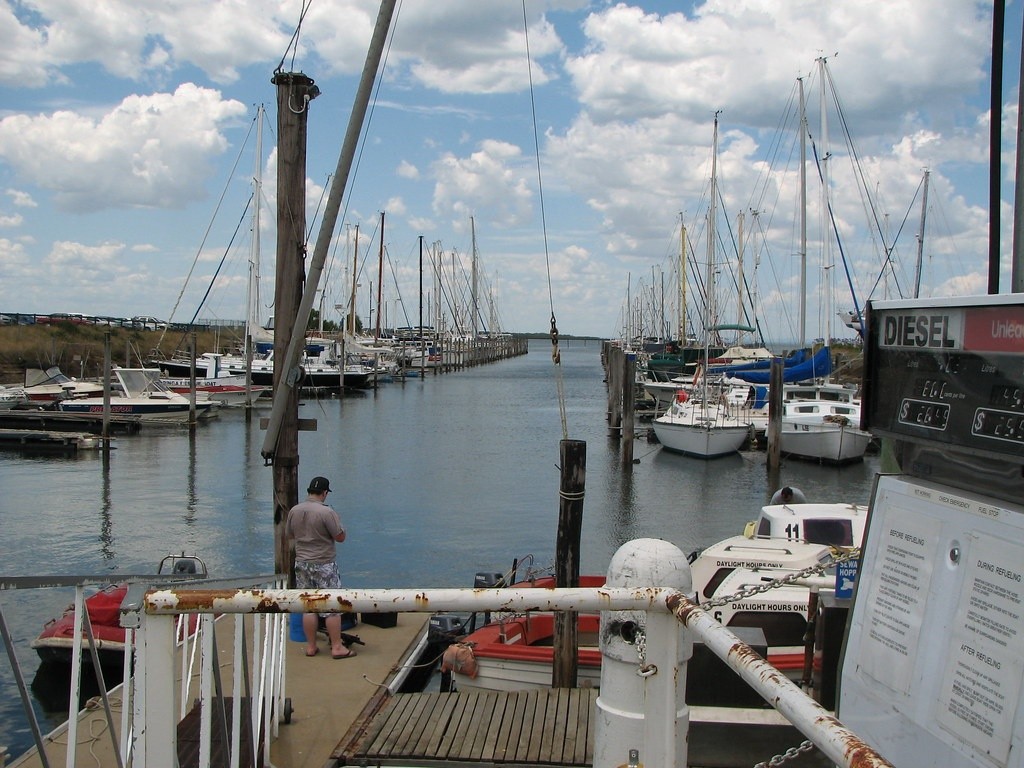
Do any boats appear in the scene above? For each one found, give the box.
[30,549,223,674]
[475,501,869,613]
[443,536,854,691]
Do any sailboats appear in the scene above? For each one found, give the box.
[0,101,501,428]
[618,54,934,466]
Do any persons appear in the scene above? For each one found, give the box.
[284,476,358,659]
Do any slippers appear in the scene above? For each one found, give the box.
[332,647,358,660]
[305,648,316,660]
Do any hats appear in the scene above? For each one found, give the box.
[307,477,333,495]
